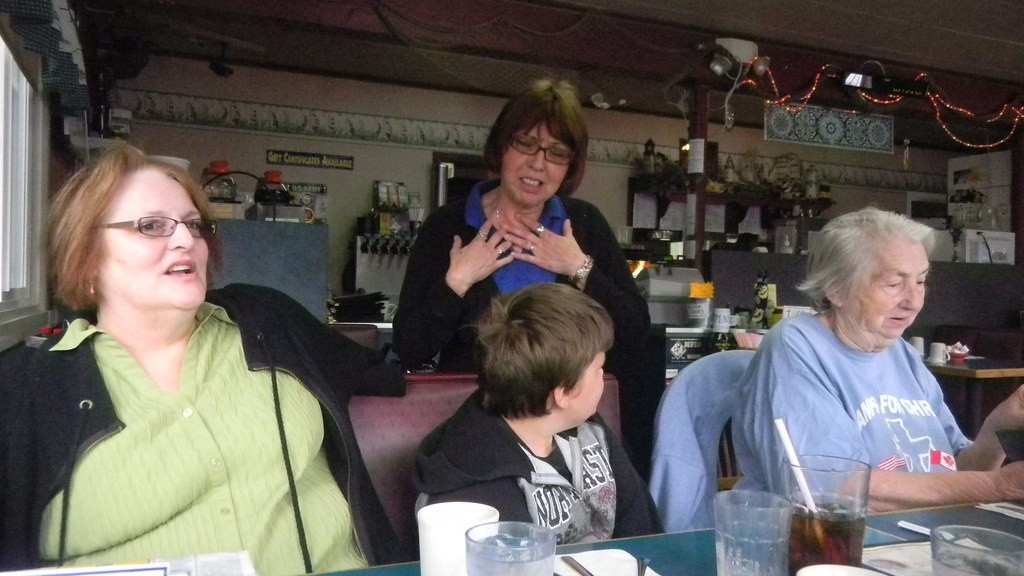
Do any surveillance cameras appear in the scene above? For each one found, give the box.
[210,61,233,76]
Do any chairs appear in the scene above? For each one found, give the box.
[648,350,757,534]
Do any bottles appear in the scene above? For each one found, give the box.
[198,160,238,203]
[254,169,291,206]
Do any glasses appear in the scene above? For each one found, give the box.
[97,216,217,238]
[509,134,576,166]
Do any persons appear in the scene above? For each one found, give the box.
[391,77,651,374]
[732,205,1024,515]
[409,283,664,559]
[0,143,404,576]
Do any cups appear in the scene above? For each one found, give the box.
[712,489,794,576]
[376,301,397,321]
[952,208,999,230]
[712,308,731,333]
[781,453,872,576]
[417,501,499,576]
[929,342,951,365]
[465,520,557,576]
[930,524,1024,576]
[909,336,925,362]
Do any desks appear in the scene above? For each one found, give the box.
[918,355,1024,442]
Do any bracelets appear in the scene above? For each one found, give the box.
[568,253,595,283]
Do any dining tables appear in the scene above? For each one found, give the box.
[293,498,1024,576]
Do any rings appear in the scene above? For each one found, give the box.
[495,246,504,254]
[475,229,488,239]
[528,242,535,253]
[535,224,544,235]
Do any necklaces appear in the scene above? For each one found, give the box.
[495,188,499,224]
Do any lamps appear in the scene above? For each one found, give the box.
[703,44,737,78]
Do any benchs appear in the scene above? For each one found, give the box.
[349,369,621,562]
[934,324,1024,468]
